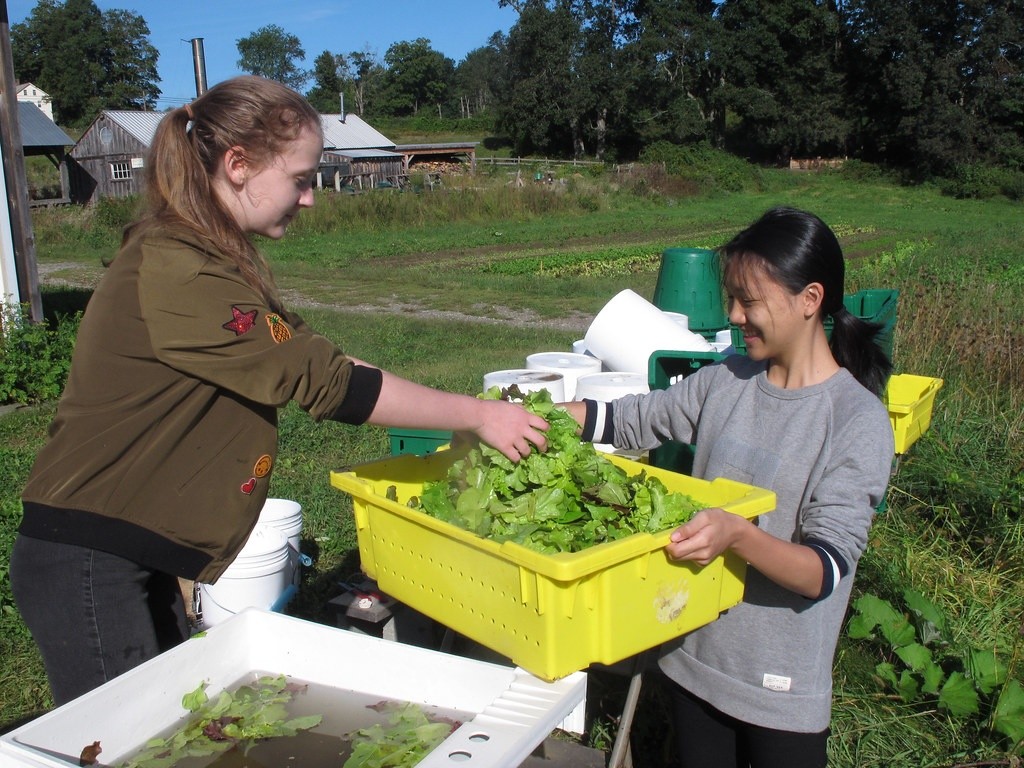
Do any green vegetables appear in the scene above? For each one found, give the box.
[78,674,465,768]
[417,383,710,555]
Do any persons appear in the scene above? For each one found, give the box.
[552,204,896,767]
[6,73,553,708]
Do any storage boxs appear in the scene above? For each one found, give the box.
[331,442,777,683]
[881,374,943,454]
[647,350,728,476]
[728,289,900,394]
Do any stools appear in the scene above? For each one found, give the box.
[326,580,435,649]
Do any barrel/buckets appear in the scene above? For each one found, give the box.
[201,527,296,635]
[257,499,312,584]
[485,289,733,453]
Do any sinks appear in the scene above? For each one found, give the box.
[12,618,517,767]
